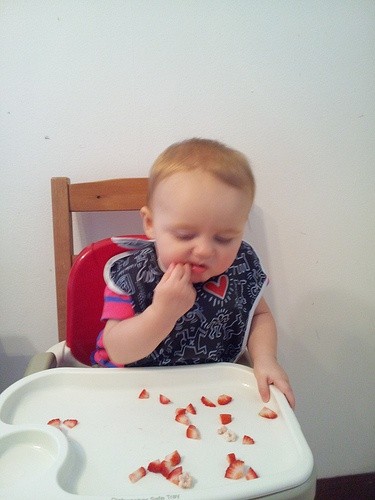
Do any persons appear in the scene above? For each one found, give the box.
[93,139,297,410]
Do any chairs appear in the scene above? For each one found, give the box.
[22,176,315,500]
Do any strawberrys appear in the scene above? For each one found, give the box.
[47,418,60,428]
[63,420,78,428]
[129,389,278,488]
